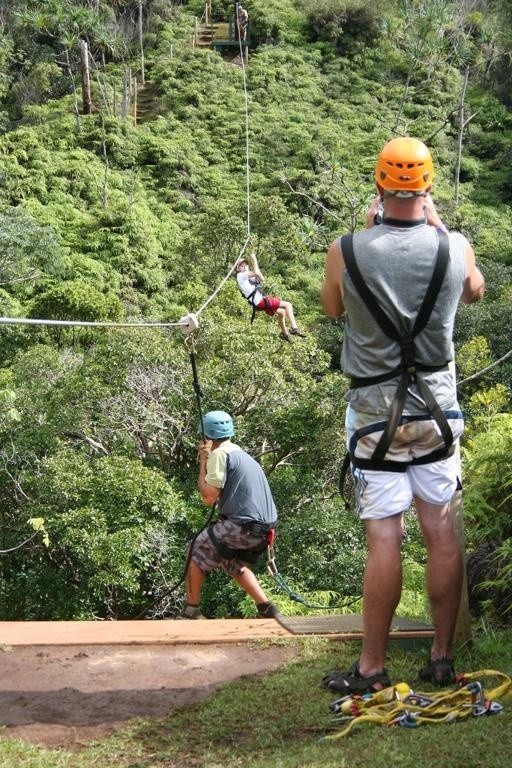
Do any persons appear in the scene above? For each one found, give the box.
[318,134,489,694]
[233,248,307,344]
[162,410,280,619]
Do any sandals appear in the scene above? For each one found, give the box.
[418,658,457,688]
[322,658,391,696]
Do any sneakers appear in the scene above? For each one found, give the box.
[197,411,235,439]
[279,332,295,344]
[164,603,202,620]
[289,327,307,337]
[256,601,281,618]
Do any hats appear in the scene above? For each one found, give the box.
[235,259,249,271]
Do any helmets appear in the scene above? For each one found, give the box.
[375,137,434,199]
